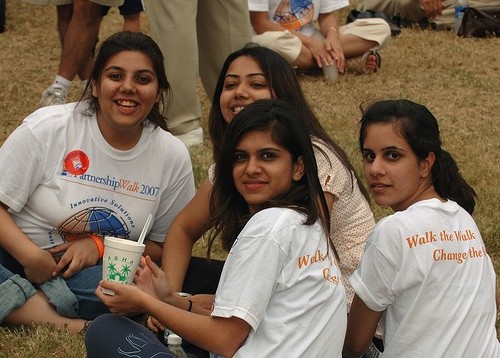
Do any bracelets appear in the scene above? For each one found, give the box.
[90,232,105,259]
[187,300,192,311]
[325,26,339,38]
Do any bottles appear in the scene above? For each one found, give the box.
[455,0,468,33]
[168,334,187,358]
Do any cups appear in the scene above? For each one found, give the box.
[102,236,146,295]
[323,60,338,81]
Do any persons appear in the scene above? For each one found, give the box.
[148,42,383,358]
[84,98,348,358]
[348,0,500,31]
[143,0,253,146]
[343,99,500,358]
[0,31,196,336]
[92,0,146,59]
[247,0,392,75]
[24,0,125,108]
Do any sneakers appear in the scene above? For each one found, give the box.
[173,126,205,148]
[347,10,401,36]
[39,87,68,106]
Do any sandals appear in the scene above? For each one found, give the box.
[345,51,382,76]
